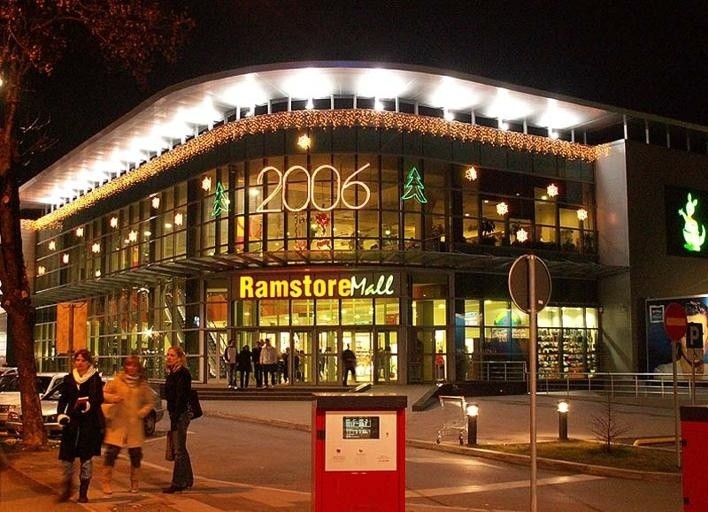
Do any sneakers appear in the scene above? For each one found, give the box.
[228,383,276,390]
[163,483,192,494]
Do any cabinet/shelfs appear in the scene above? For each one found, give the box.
[534,329,599,380]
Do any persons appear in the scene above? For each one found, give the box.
[225,336,332,390]
[161,344,194,494]
[436,350,444,379]
[100,353,155,496]
[342,343,359,385]
[55,349,105,503]
[653,300,707,382]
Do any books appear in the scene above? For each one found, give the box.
[73,396,90,410]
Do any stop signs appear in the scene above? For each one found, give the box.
[663,303,688,342]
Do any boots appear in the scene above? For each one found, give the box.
[101,467,114,496]
[130,467,140,494]
[58,478,90,503]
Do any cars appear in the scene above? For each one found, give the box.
[0,365,167,438]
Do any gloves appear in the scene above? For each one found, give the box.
[59,418,70,426]
[78,400,87,411]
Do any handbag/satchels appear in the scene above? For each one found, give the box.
[100,402,117,420]
[187,389,203,421]
[165,430,174,461]
